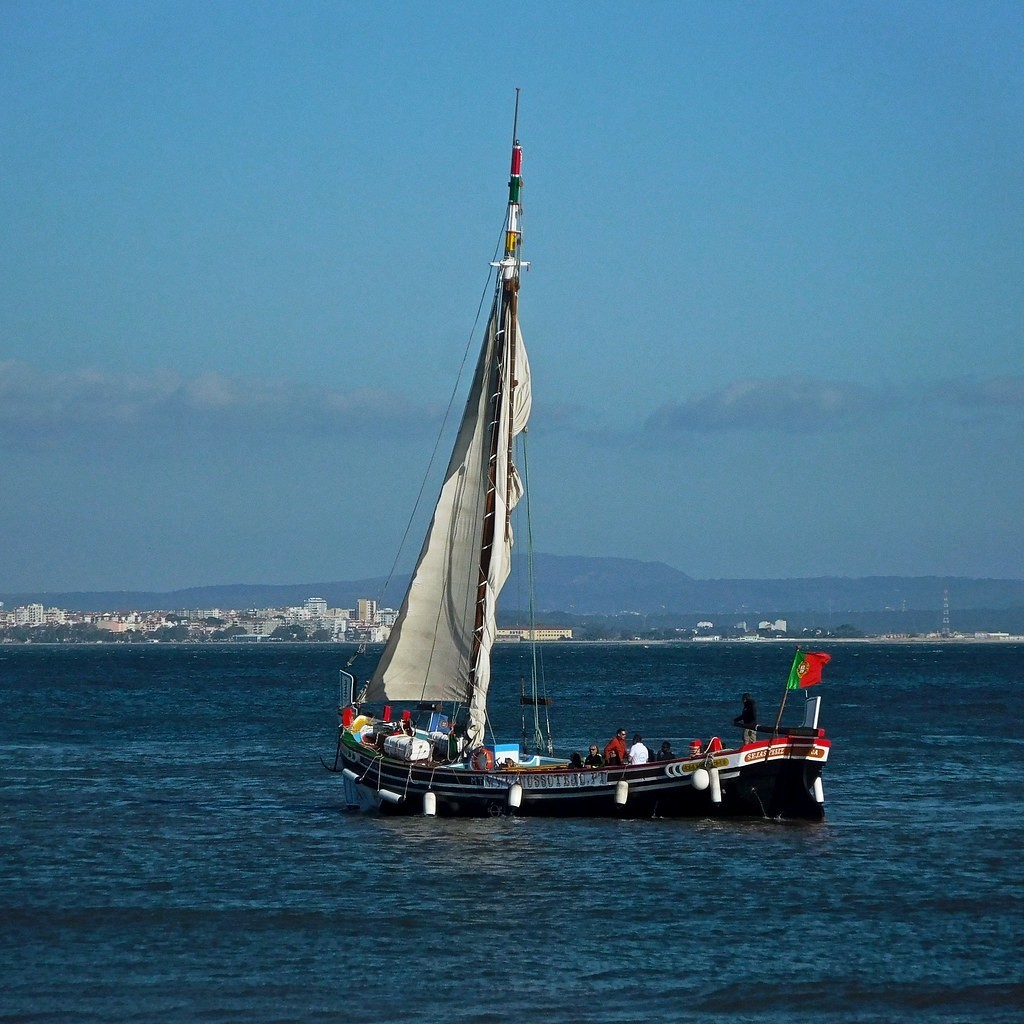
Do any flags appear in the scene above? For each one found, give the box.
[786,650,830,689]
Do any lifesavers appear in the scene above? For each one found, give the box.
[470,748,491,770]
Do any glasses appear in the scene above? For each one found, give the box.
[621,734,626,736]
[589,749,597,751]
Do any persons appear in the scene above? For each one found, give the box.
[504,758,519,768]
[734,694,757,745]
[568,729,676,770]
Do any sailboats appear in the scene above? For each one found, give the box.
[326,79,836,821]
